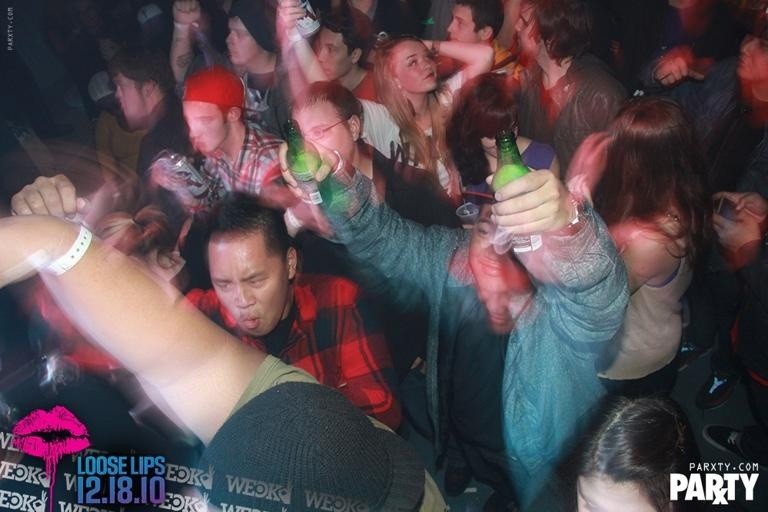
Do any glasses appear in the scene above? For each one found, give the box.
[303,116,355,141]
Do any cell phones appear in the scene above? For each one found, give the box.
[716,198,740,222]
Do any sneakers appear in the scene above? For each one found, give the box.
[703,423,747,461]
[690,361,743,412]
[673,334,712,369]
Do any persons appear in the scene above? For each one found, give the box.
[275,141,629,512]
[108,43,201,200]
[8,174,214,308]
[449,3,530,91]
[574,392,708,511]
[446,72,561,189]
[290,79,465,226]
[638,0,728,93]
[703,192,764,469]
[566,97,706,403]
[313,9,377,101]
[512,4,630,172]
[182,69,302,209]
[168,2,299,145]
[668,21,765,411]
[87,70,151,186]
[284,4,492,203]
[10,173,403,427]
[0,215,448,511]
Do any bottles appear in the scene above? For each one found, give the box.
[169,155,211,202]
[285,119,330,208]
[285,1,321,38]
[492,130,544,262]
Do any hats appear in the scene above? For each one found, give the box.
[229,1,282,54]
[181,66,247,115]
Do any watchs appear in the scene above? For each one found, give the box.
[40,218,94,277]
[544,193,585,237]
